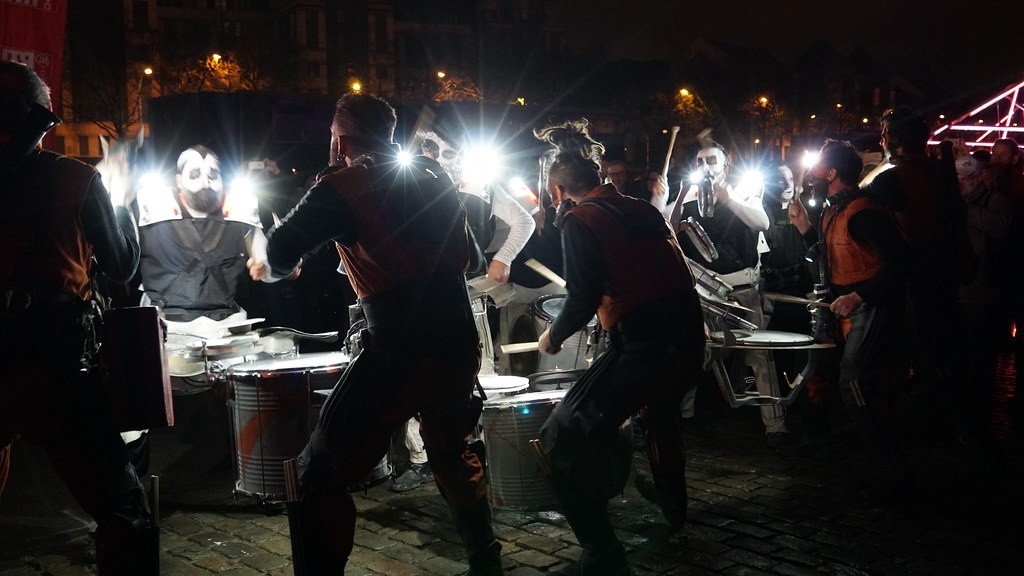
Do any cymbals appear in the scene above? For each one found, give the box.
[719,300,755,313]
[211,317,266,335]
[528,368,590,384]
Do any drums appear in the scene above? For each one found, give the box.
[686,216,719,261]
[478,374,529,401]
[146,337,297,508]
[350,296,494,464]
[710,331,813,347]
[698,178,715,216]
[699,299,758,331]
[532,292,610,372]
[482,388,571,513]
[224,352,394,506]
[686,257,734,305]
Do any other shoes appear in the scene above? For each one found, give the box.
[766,431,791,466]
[799,431,833,460]
[547,559,634,576]
[635,473,657,499]
[456,540,504,576]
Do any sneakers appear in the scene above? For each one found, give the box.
[391,461,436,492]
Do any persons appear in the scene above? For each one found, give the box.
[264,93,506,576]
[536,115,706,576]
[788,108,974,467]
[0,60,176,576]
[96,127,802,497]
[929,139,1024,304]
